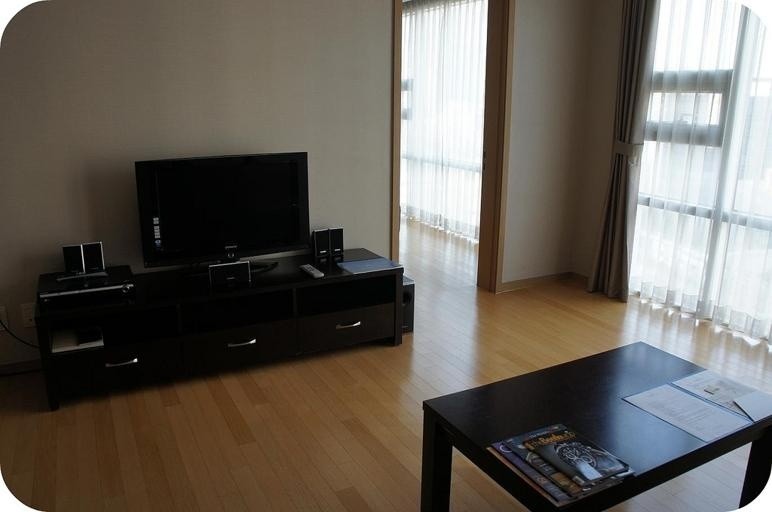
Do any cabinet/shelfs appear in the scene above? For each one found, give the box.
[35,248,404,412]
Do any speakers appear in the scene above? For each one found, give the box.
[402,275,414,333]
[329,227,344,256]
[208,260,250,288]
[310,229,330,258]
[82,241,105,273]
[62,246,84,275]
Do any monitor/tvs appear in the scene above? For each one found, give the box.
[135,152,310,277]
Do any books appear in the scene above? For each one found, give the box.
[483,447,623,507]
[524,427,630,486]
[502,422,638,496]
[77,329,104,344]
[492,440,623,501]
[51,327,105,353]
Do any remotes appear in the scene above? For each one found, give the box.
[299,263,325,278]
